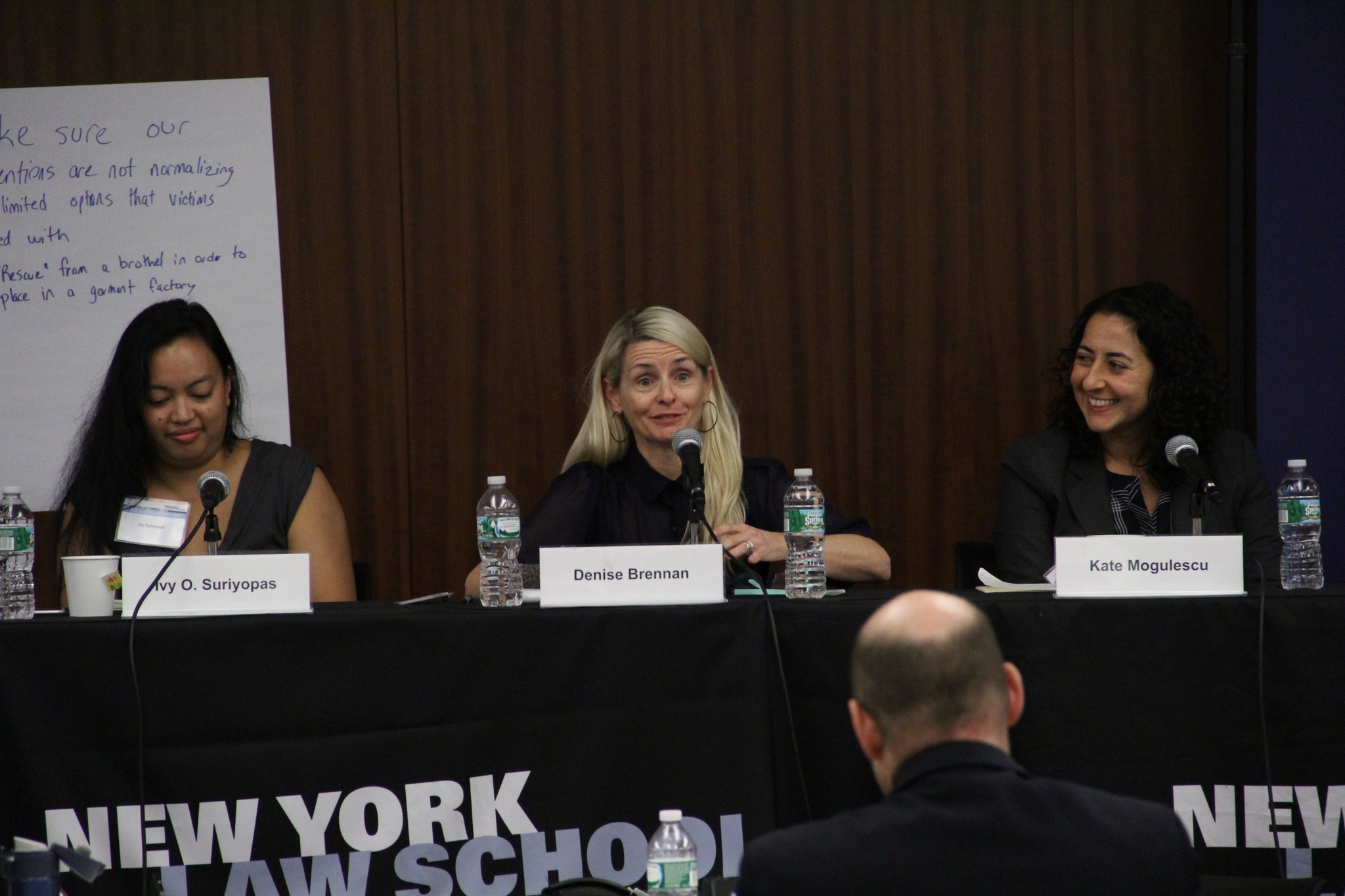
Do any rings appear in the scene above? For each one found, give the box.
[743,541,754,553]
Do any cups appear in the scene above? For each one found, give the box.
[60,555,121,617]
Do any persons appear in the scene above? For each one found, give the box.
[736,591,1197,894]
[995,282,1278,585]
[462,305,890,603]
[52,297,355,604]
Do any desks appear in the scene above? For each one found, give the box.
[0,571,1345,896]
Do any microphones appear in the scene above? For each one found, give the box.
[196,470,232,510]
[1165,435,1226,509]
[671,427,706,518]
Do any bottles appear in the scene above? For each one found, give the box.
[1277,460,1324,589]
[476,475,524,608]
[646,809,698,896]
[784,468,827,598]
[0,486,35,620]
